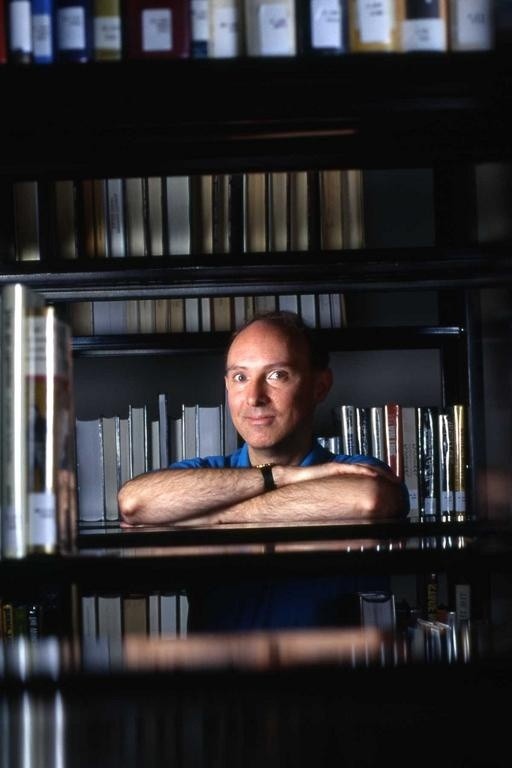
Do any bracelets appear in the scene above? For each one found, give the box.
[255,463,280,496]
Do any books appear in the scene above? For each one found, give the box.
[1,1,510,58]
[75,393,466,528]
[1,170,491,264]
[63,292,345,332]
[82,596,187,652]
[356,584,468,669]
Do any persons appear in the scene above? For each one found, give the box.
[119,312,409,662]
